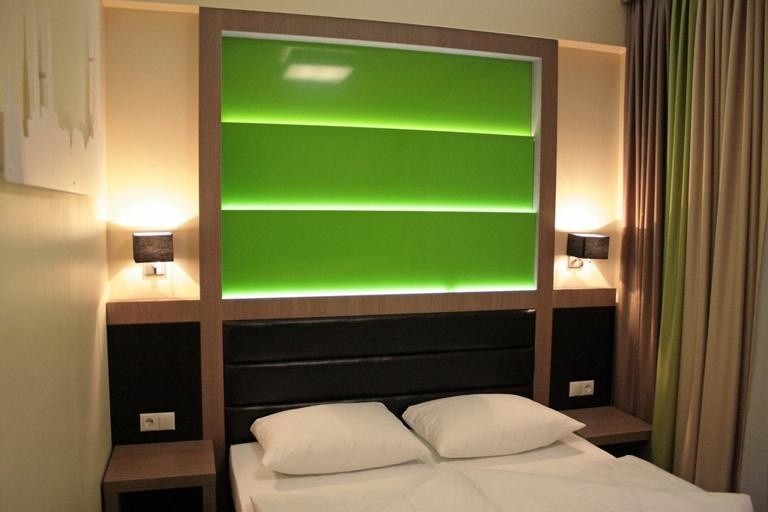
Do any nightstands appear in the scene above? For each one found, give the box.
[103,438,218,512]
[555,405,653,446]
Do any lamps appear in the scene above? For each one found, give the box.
[567,234,609,268]
[133,232,174,275]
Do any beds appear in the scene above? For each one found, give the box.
[228,427,754,512]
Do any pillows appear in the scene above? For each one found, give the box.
[402,393,588,459]
[250,400,426,476]
